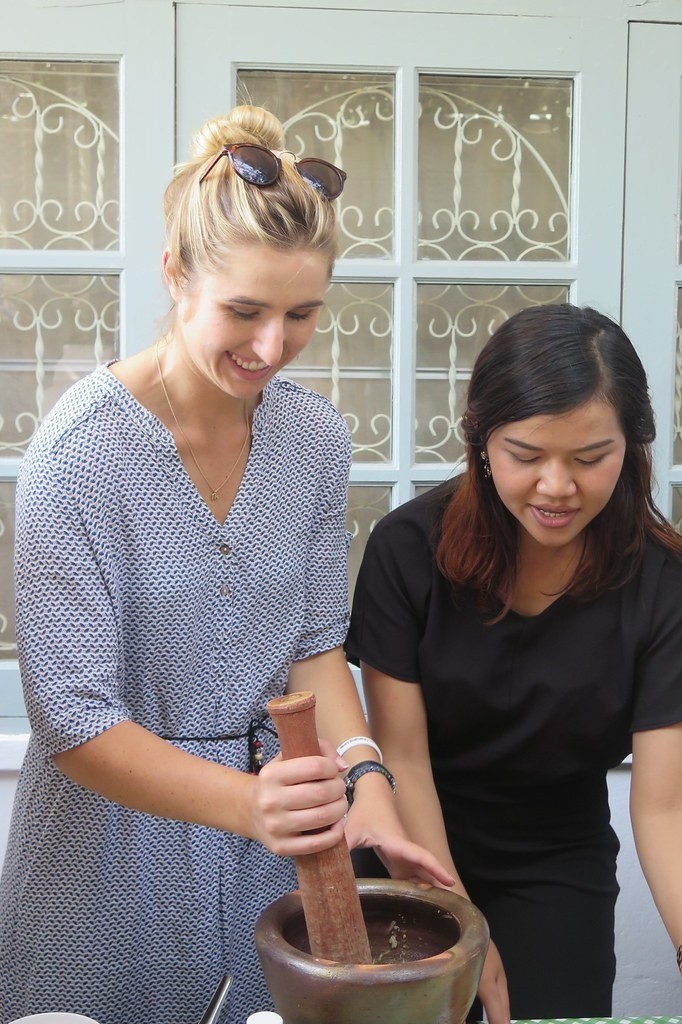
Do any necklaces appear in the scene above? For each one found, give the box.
[154,342,250,501]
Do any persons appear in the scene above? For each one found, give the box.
[1,104,456,1024]
[342,303,682,1024]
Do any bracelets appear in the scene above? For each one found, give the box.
[342,760,398,805]
[337,737,384,766]
[677,944,682,974]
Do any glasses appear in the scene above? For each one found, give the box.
[200,142,347,202]
